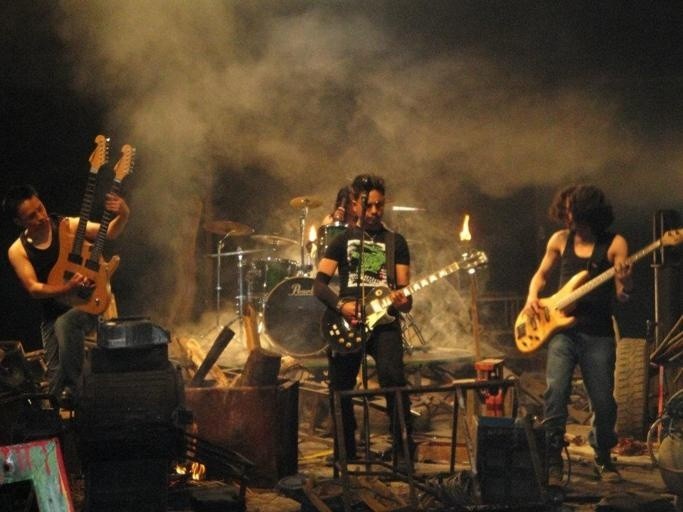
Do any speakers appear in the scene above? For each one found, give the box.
[476,424,550,512]
[75,362,186,512]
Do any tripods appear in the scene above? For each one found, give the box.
[198,241,241,346]
[206,245,274,350]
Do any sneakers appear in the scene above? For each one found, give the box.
[396,449,414,475]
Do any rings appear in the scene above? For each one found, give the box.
[81,282,84,290]
[336,215,338,219]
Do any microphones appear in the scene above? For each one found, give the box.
[359,174,371,204]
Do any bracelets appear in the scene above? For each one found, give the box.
[622,287,632,296]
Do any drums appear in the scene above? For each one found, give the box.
[243,235,312,287]
[262,275,338,358]
[316,221,352,269]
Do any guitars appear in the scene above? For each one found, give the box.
[320,249,488,354]
[515,228,683,353]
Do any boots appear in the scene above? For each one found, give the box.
[593,446,622,483]
[547,448,563,487]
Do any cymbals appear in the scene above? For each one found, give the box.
[205,220,254,237]
[291,196,324,209]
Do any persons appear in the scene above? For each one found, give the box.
[521,183,632,485]
[311,174,416,477]
[321,184,354,227]
[4,182,130,410]
